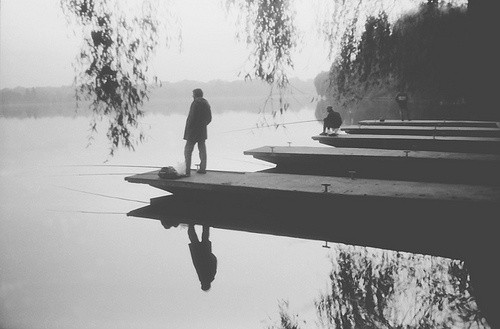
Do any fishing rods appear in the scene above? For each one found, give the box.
[64,163,167,177]
[242,116,324,129]
[46,207,129,215]
[57,184,151,206]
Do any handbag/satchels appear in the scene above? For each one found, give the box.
[157,166,178,179]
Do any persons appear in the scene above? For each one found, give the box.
[396,87,411,121]
[180,88,212,176]
[319,106,342,136]
